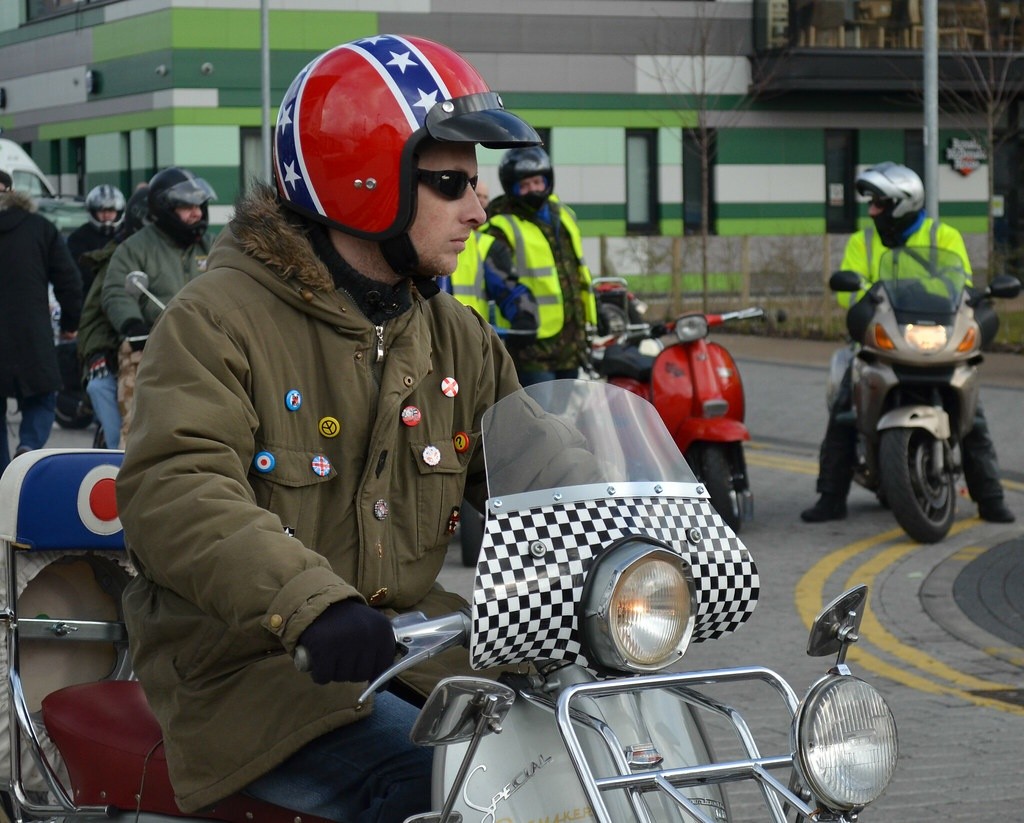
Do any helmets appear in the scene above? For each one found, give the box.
[855,161,925,218]
[273,33,544,241]
[147,167,219,242]
[84,183,127,237]
[128,182,157,235]
[499,146,553,209]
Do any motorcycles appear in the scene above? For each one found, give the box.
[829,244,1022,545]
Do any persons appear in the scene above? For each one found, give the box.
[78,164,218,450]
[451,146,599,413]
[803,162,1018,525]
[52,184,127,345]
[117,36,608,822]
[0,170,82,481]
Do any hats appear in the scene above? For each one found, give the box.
[0,170,12,193]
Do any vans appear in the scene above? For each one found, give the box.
[0,137,64,234]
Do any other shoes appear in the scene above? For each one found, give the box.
[977,499,1016,524]
[799,493,847,523]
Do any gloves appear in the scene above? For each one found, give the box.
[298,600,399,684]
[88,355,109,381]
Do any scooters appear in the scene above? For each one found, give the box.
[49,312,100,431]
[591,305,766,535]
[592,275,648,337]
[0,377,897,823]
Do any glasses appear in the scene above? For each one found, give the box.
[419,168,478,200]
[865,196,891,209]
